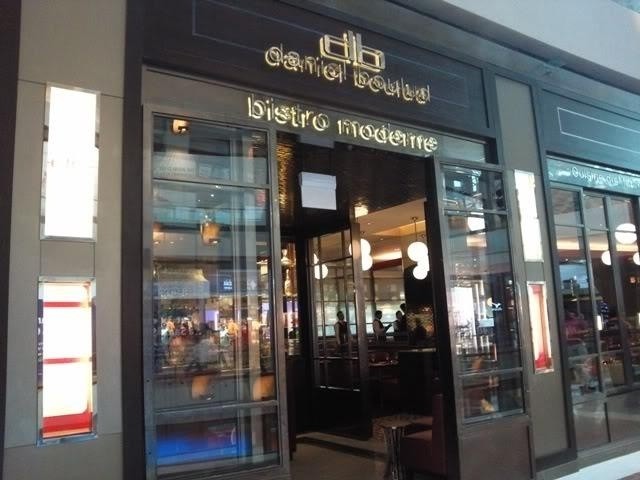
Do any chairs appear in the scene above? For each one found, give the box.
[398,377,446,479]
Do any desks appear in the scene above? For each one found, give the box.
[373,415,423,480]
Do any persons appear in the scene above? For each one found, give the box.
[154,320,227,373]
[602,307,634,350]
[393,303,407,341]
[289,327,297,339]
[333,311,357,352]
[565,310,592,383]
[372,311,392,341]
